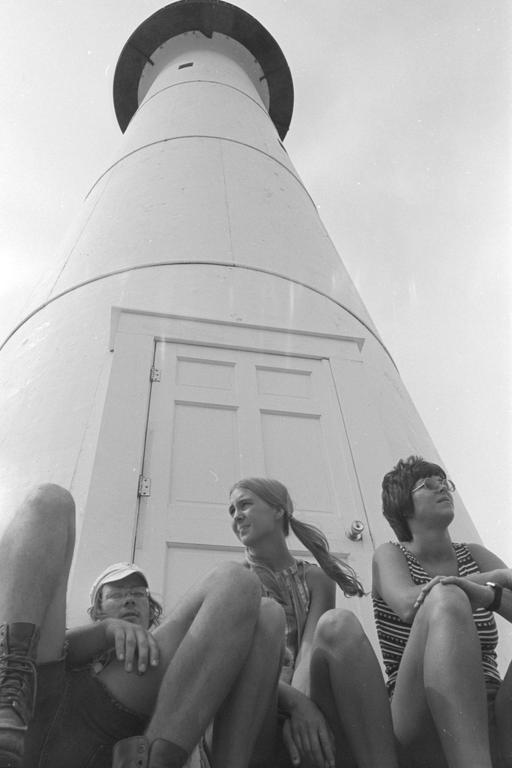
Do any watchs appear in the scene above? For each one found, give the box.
[482,581,503,612]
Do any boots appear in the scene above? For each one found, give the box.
[111,735,189,768]
[0,621,41,768]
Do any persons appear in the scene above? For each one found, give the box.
[200,474,400,768]
[372,455,512,767]
[0,480,263,768]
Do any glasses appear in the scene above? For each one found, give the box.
[105,585,150,603]
[409,478,455,494]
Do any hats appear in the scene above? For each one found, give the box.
[89,561,149,608]
